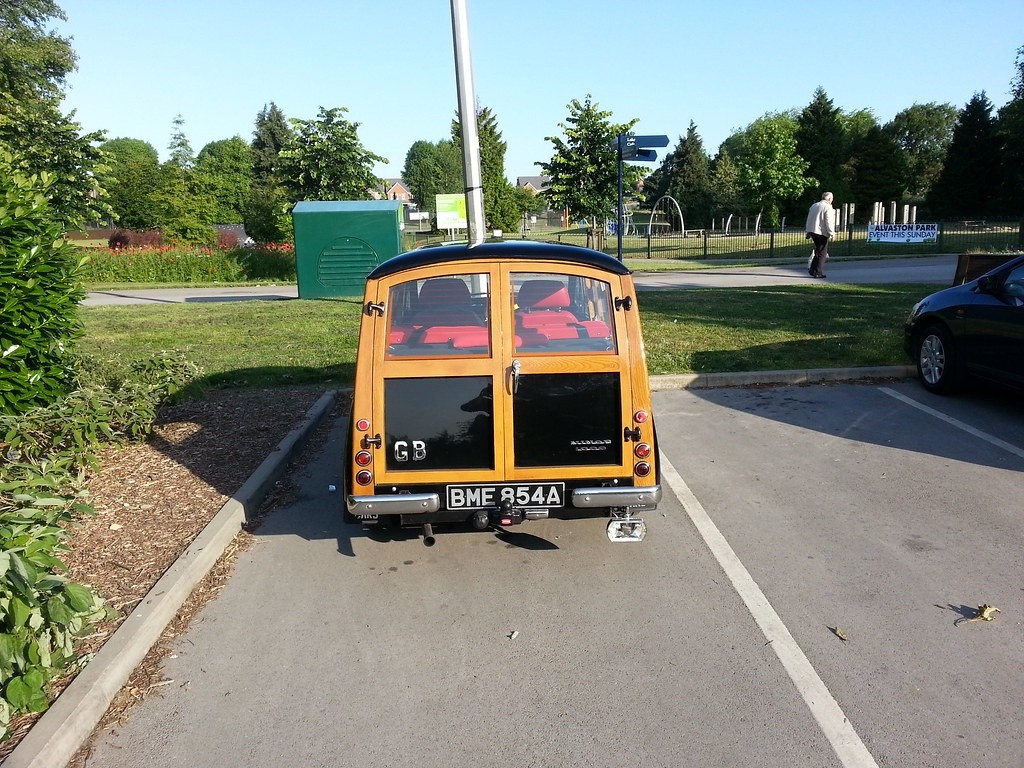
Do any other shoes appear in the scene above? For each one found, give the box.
[808,270,816,277]
[815,274,826,278]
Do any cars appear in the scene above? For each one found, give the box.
[902,254,1024,394]
[344,237,664,545]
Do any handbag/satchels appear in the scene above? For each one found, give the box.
[808,249,830,269]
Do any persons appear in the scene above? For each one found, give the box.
[806,192,835,279]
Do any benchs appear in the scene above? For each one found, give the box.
[389,321,610,348]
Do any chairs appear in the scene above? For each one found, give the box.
[514,280,578,325]
[411,279,479,325]
